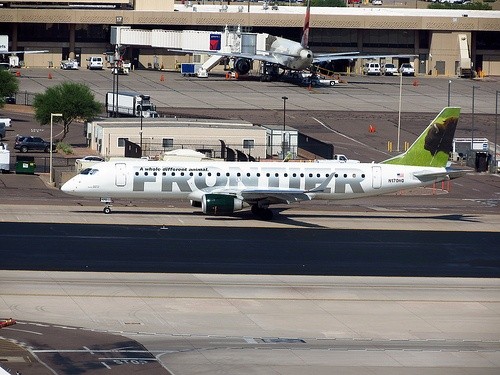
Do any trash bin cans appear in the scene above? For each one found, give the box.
[15,155,36,174]
[476,152,490,172]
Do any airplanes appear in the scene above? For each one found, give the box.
[167,33,420,83]
[60,106,475,222]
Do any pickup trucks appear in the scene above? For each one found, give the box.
[380,63,399,76]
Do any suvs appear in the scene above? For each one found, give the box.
[13,136,57,153]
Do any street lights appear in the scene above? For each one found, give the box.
[114,59,122,117]
[281,96,289,160]
[136,94,146,147]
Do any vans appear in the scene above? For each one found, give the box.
[362,62,381,76]
[86,56,103,70]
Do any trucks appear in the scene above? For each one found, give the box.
[105,92,161,118]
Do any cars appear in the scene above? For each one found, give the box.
[458,68,476,78]
[60,59,79,70]
[111,66,129,75]
[419,0,474,6]
[348,0,383,5]
[3,93,16,104]
[398,62,415,76]
[289,71,340,87]
[0,116,12,174]
